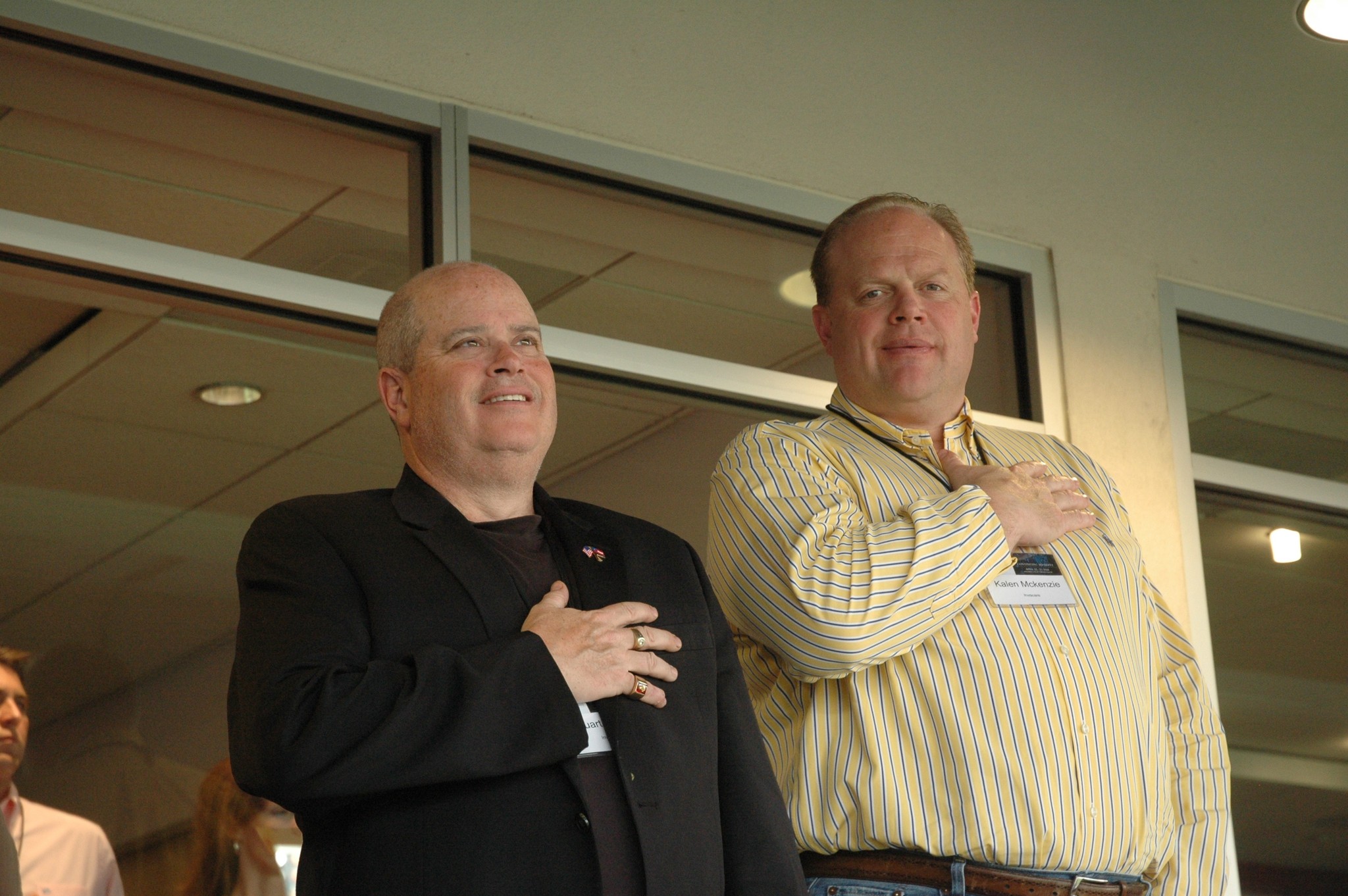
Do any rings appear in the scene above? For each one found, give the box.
[631,674,648,700]
[629,628,645,650]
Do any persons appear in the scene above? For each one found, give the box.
[182,756,304,896]
[0,646,126,895]
[226,263,809,896]
[705,192,1233,896]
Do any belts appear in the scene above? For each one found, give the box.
[801,846,1150,896]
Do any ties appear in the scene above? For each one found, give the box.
[0,812,23,896]
